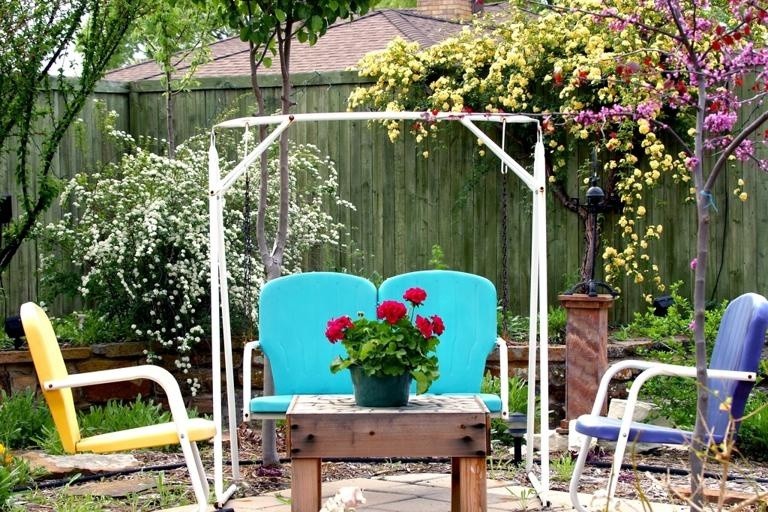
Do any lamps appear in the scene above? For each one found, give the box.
[567,183,620,296]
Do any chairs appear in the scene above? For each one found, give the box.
[241,272,378,422]
[569,292,764,512]
[20,302,218,510]
[377,270,510,420]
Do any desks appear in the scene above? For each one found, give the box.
[286,395,489,512]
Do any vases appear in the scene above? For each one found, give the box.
[347,363,411,406]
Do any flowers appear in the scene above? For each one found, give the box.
[325,288,444,396]
